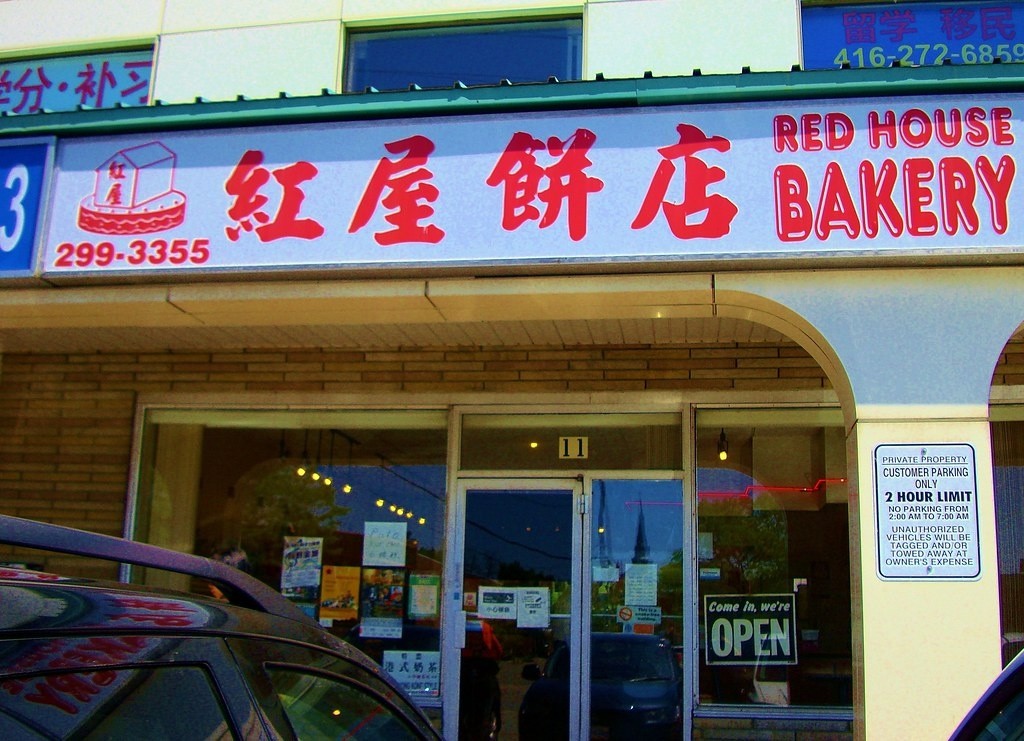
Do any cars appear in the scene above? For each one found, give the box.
[947,642,1024,741]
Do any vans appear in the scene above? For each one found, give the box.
[514,629,694,740]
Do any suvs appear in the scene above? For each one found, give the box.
[1,509,445,741]
[339,607,504,740]
